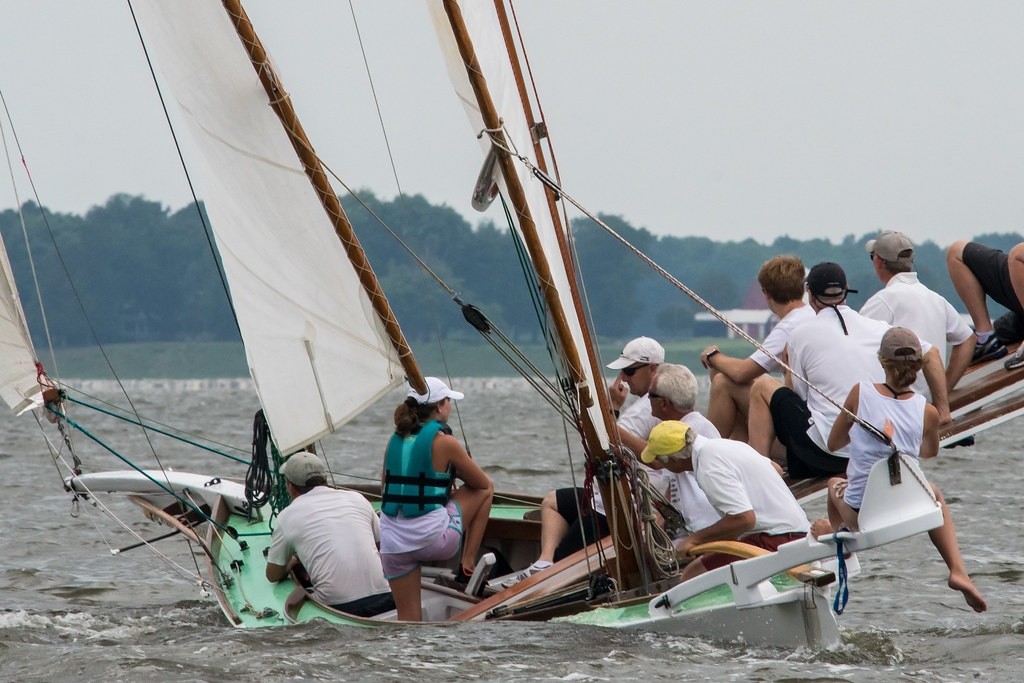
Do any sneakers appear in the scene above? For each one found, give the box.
[501,562,553,587]
[1004,340,1024,370]
[968,329,1007,366]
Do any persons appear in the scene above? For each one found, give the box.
[700,254,816,443]
[946,240,1024,369]
[641,420,812,584]
[580,364,724,536]
[501,336,664,591]
[379,377,494,621]
[810,326,988,612]
[859,230,977,404]
[265,451,396,617]
[748,262,955,478]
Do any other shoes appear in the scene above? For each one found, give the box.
[455,564,489,587]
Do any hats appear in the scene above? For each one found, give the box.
[804,262,846,297]
[877,327,923,361]
[279,452,327,487]
[406,377,464,405]
[606,336,665,369]
[865,229,914,262]
[640,420,690,464]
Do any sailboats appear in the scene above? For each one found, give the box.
[0,0,1023,657]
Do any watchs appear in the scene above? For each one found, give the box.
[706,349,720,366]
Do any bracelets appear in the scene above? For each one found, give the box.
[610,409,620,418]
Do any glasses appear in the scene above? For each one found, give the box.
[762,284,775,293]
[870,252,886,263]
[623,363,659,376]
[649,391,671,405]
